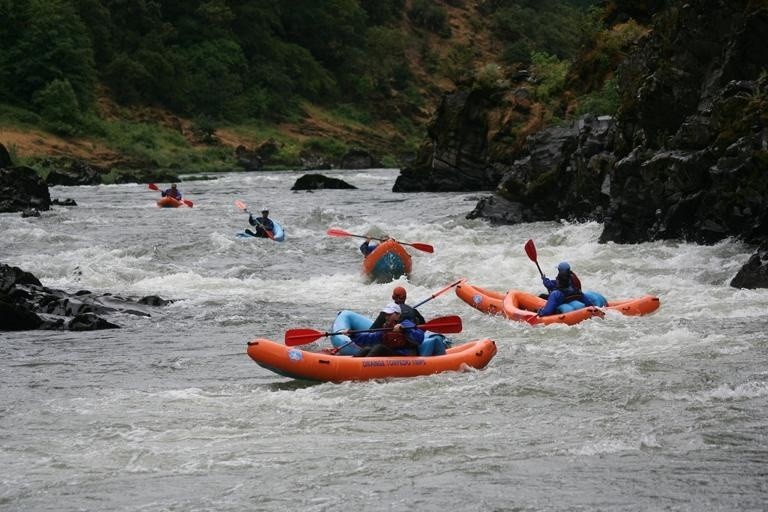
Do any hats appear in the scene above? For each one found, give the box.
[261,208,269,213]
[172,184,176,187]
[380,303,402,315]
[393,287,406,299]
[380,235,389,241]
[557,262,571,283]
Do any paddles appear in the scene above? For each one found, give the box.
[235,200,274,241]
[284,315,462,347]
[148,183,193,208]
[316,278,470,356]
[525,239,551,295]
[326,230,433,252]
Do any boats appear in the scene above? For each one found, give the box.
[235,218,284,245]
[361,224,412,283]
[454,280,660,325]
[157,195,192,210]
[245,334,498,383]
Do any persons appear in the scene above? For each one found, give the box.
[367,287,426,333]
[536,273,586,317]
[537,262,582,300]
[342,300,425,357]
[359,232,397,258]
[161,183,183,202]
[244,205,275,238]
